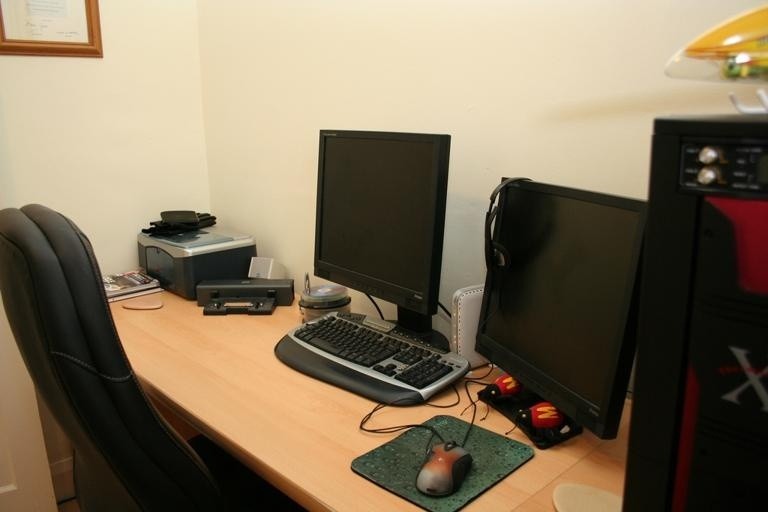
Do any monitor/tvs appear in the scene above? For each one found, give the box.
[313,130,452,353]
[474,176,650,451]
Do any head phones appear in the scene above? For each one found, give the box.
[484,176,533,272]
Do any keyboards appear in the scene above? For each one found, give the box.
[274,311,471,407]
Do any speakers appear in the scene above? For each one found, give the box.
[620,115,768,512]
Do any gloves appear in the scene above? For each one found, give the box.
[142,212,217,237]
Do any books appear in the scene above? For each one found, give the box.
[102,267,160,299]
[107,287,165,303]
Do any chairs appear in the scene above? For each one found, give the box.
[0,202,308,512]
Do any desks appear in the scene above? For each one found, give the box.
[106,288,633,512]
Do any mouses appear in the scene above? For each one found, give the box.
[415,441,473,498]
